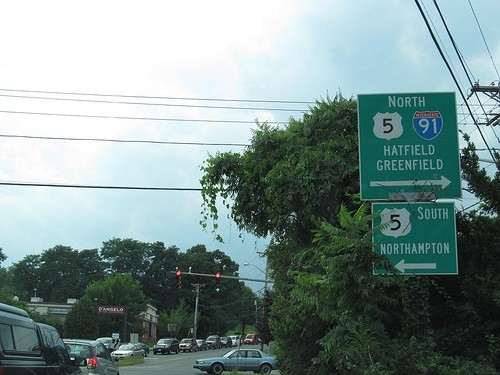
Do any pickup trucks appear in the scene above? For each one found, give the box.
[0,303,73,375]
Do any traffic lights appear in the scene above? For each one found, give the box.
[216,272,221,294]
[176,271,182,290]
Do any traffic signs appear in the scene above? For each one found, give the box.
[358,93,465,202]
[370,203,460,277]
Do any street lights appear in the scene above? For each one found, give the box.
[243,263,268,350]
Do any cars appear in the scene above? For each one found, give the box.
[194,348,279,375]
[96,334,257,362]
[62,338,120,375]
